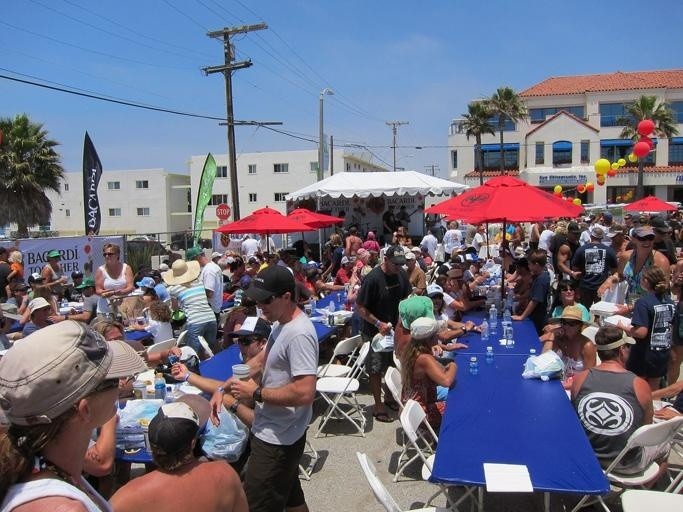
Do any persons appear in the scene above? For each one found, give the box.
[0,195,683,511]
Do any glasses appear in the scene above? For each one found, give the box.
[237,338,258,345]
[635,236,655,241]
[561,288,575,292]
[103,252,117,257]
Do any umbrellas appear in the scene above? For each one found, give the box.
[421,176,586,319]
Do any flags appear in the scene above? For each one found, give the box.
[83,130,102,235]
[193,154,218,247]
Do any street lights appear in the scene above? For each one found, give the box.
[316,79,336,181]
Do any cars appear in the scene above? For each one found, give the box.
[126,236,167,250]
[170,233,214,251]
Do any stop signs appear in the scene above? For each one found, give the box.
[215,203,233,221]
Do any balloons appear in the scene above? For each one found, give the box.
[607,169,617,178]
[637,137,654,151]
[552,175,605,208]
[628,154,638,162]
[594,159,611,175]
[610,163,619,170]
[632,143,651,158]
[617,159,627,166]
[638,121,657,137]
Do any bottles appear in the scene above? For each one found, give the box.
[309,297,316,315]
[529,349,536,359]
[64,297,69,308]
[154,371,167,401]
[327,291,352,327]
[163,385,174,404]
[467,262,515,377]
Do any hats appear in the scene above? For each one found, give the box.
[0,319,151,425]
[160,258,201,287]
[228,315,272,339]
[398,295,436,328]
[186,233,284,264]
[150,401,201,450]
[595,329,636,352]
[410,317,437,339]
[245,265,296,303]
[10,249,156,316]
[406,224,486,297]
[568,211,613,240]
[649,218,671,233]
[552,305,593,326]
[633,224,656,237]
[385,245,407,265]
[341,248,371,269]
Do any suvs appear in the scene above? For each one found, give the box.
[125,241,183,271]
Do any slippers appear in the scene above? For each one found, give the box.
[371,410,394,422]
[385,399,399,411]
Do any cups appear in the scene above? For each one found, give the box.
[231,364,251,382]
[142,430,153,454]
[131,382,147,400]
[136,316,145,326]
[304,304,313,318]
